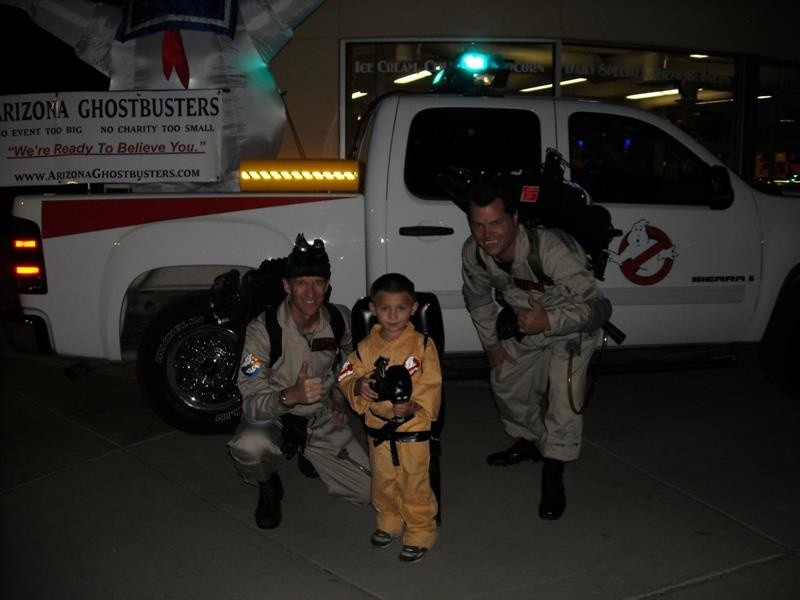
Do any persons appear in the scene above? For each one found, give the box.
[339,273,444,562]
[228,249,373,530]
[461,180,613,519]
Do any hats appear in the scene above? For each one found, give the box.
[285,239,331,276]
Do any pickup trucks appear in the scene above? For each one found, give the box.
[8,91,799,435]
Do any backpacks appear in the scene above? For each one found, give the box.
[474,149,622,286]
[245,258,345,367]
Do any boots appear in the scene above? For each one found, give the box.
[538,457,568,521]
[485,437,539,468]
[252,470,282,530]
[298,454,321,480]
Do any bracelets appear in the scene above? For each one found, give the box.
[278,389,287,406]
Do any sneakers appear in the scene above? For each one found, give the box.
[397,545,428,564]
[371,526,396,548]
